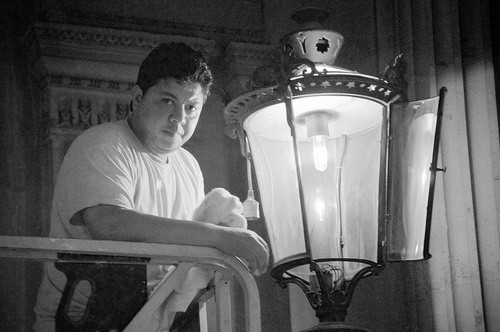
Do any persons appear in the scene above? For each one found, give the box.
[32,43,270,332]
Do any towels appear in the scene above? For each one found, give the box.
[147,188,248,332]
